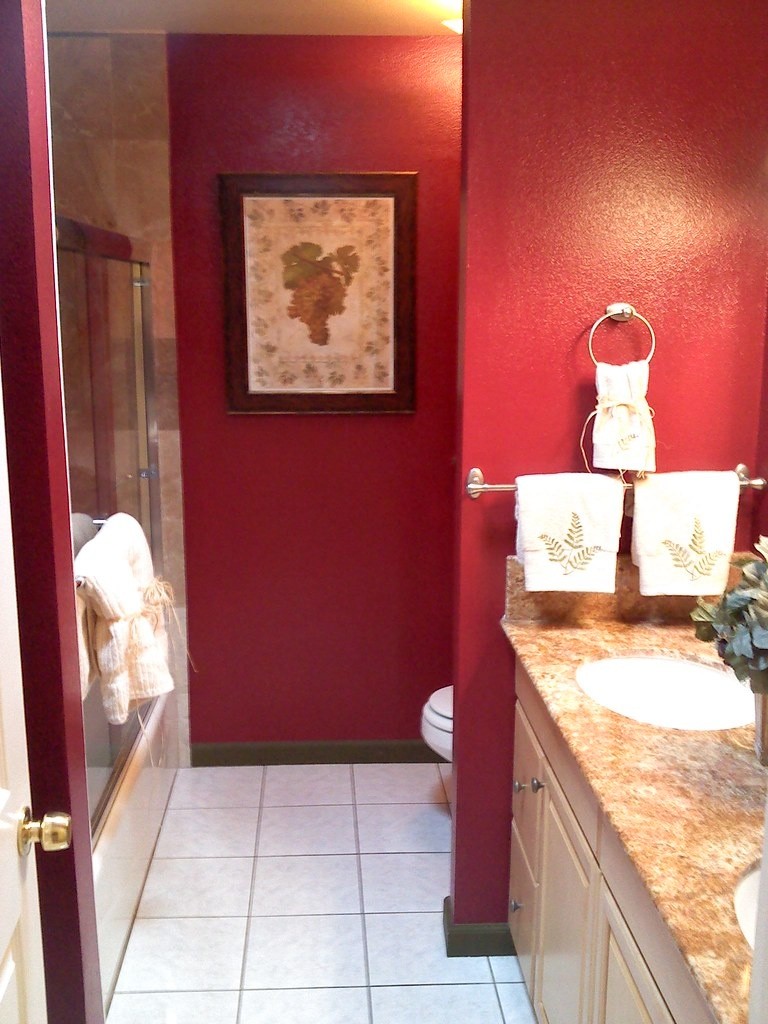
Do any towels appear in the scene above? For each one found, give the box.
[630,469,740,597]
[71,512,99,560]
[514,472,624,595]
[590,360,657,473]
[74,513,175,726]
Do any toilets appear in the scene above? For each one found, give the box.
[421,684,453,762]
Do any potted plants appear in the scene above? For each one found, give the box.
[688,531,768,768]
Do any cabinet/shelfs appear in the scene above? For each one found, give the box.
[506,652,717,1024]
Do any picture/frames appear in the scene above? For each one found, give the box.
[218,171,416,416]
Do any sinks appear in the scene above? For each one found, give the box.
[573,647,756,732]
[732,854,761,951]
